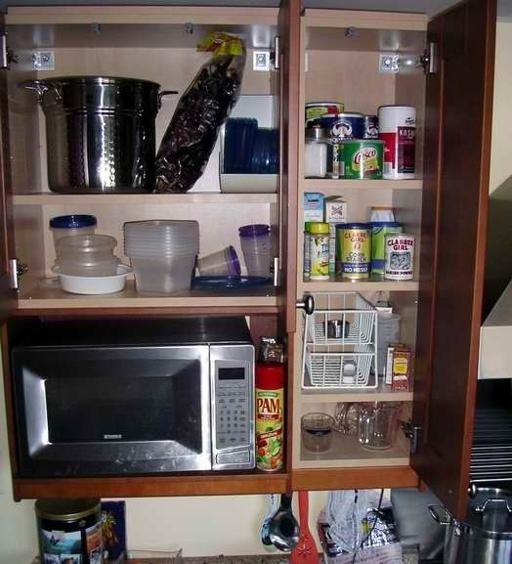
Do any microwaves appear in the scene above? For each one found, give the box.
[9,317,257,477]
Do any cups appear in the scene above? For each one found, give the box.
[302,413,334,453]
[359,401,402,451]
[197,223,272,280]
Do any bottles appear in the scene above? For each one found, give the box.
[308,223,331,280]
[367,206,396,223]
[254,359,286,471]
[304,223,311,278]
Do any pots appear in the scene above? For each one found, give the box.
[16,74,178,194]
[426,496,511,564]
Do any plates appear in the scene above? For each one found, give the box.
[222,116,282,175]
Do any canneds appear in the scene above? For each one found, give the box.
[337,138,385,180]
[32,496,107,564]
[317,112,365,180]
[305,101,346,132]
[361,114,379,140]
